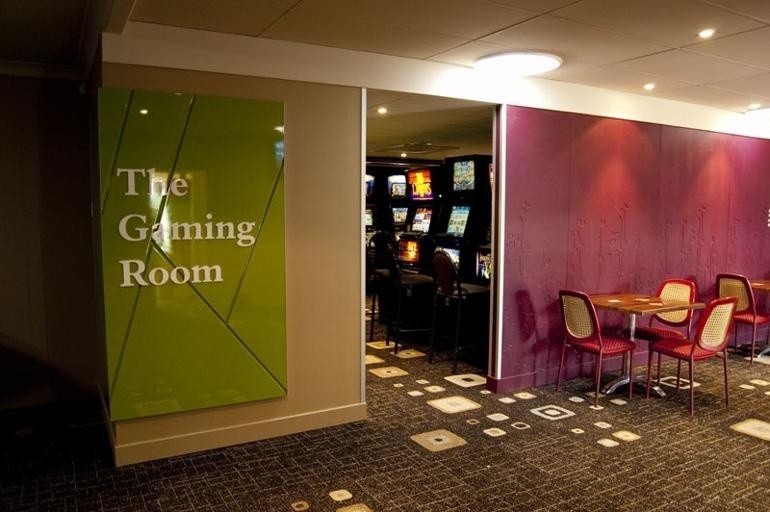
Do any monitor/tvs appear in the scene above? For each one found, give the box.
[392,207,409,224]
[365,208,374,226]
[366,172,376,197]
[411,208,433,233]
[406,169,434,201]
[452,160,476,191]
[386,174,407,197]
[474,247,491,279]
[434,247,461,270]
[397,241,421,265]
[446,205,472,238]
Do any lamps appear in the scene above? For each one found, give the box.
[472,51,566,81]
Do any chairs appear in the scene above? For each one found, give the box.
[367,229,438,354]
[716,272,769,367]
[620,277,697,402]
[555,287,635,408]
[645,295,740,413]
[428,249,490,376]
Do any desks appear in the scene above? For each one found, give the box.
[583,291,706,395]
[745,276,769,346]
